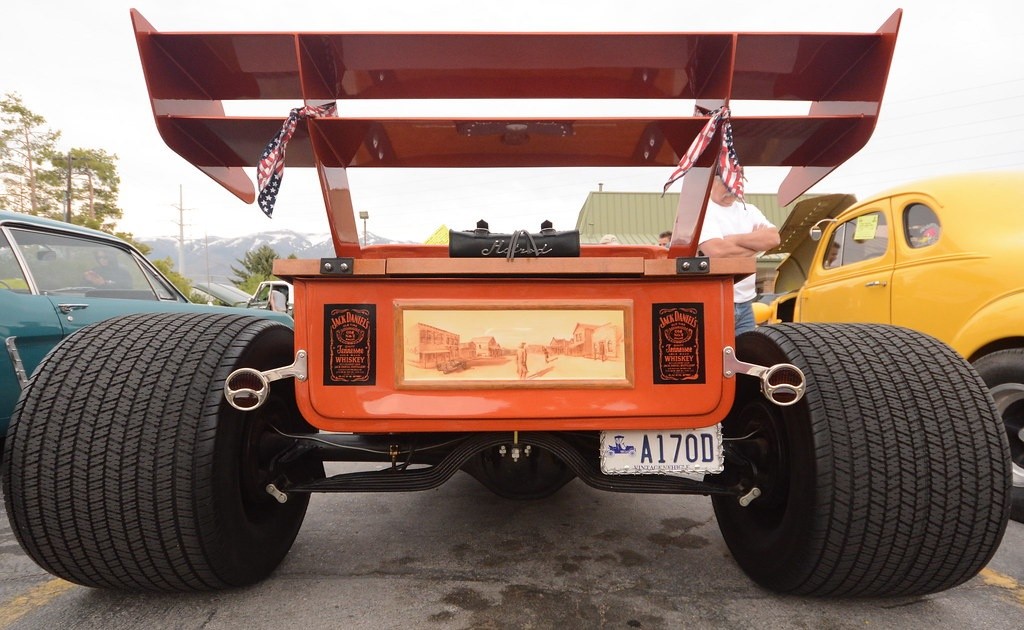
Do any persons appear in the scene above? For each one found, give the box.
[268,287,287,315]
[695,165,781,340]
[84,244,131,290]
[659,231,672,250]
[823,242,840,269]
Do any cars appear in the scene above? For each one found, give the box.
[751,168,1024,523]
[0,210,195,466]
[192,281,294,322]
